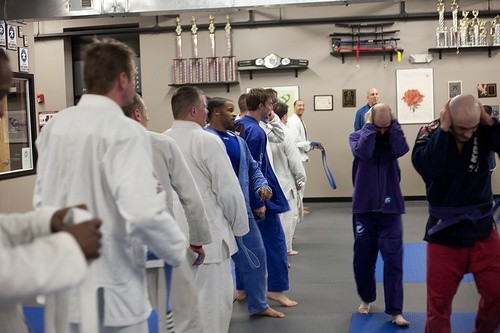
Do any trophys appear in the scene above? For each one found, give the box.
[189,16,203,83]
[450,0,461,48]
[173,17,187,83]
[435,0,448,48]
[221,14,236,82]
[206,14,220,83]
[459,9,500,46]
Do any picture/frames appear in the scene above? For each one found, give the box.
[244,85,300,118]
[313,95,334,111]
[448,80,463,99]
[341,89,357,107]
[0,72,43,180]
[394,66,435,124]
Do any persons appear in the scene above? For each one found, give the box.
[482,105,496,175]
[0,48,103,333]
[161,86,250,333]
[286,99,324,214]
[204,87,307,319]
[121,92,205,333]
[354,88,380,132]
[411,94,500,333]
[349,103,410,326]
[32,36,186,333]
